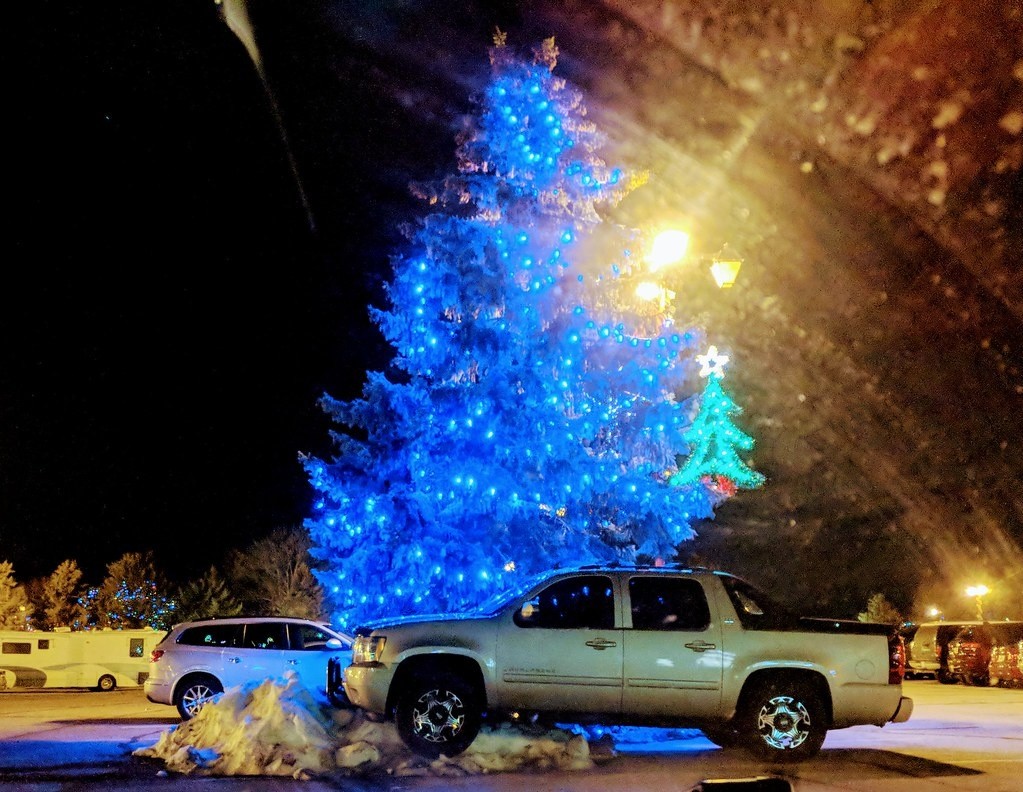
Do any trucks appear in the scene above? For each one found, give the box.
[1,624,165,693]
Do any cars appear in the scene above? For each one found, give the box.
[139,620,349,718]
[896,620,1023,688]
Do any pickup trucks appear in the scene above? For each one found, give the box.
[342,560,913,758]
